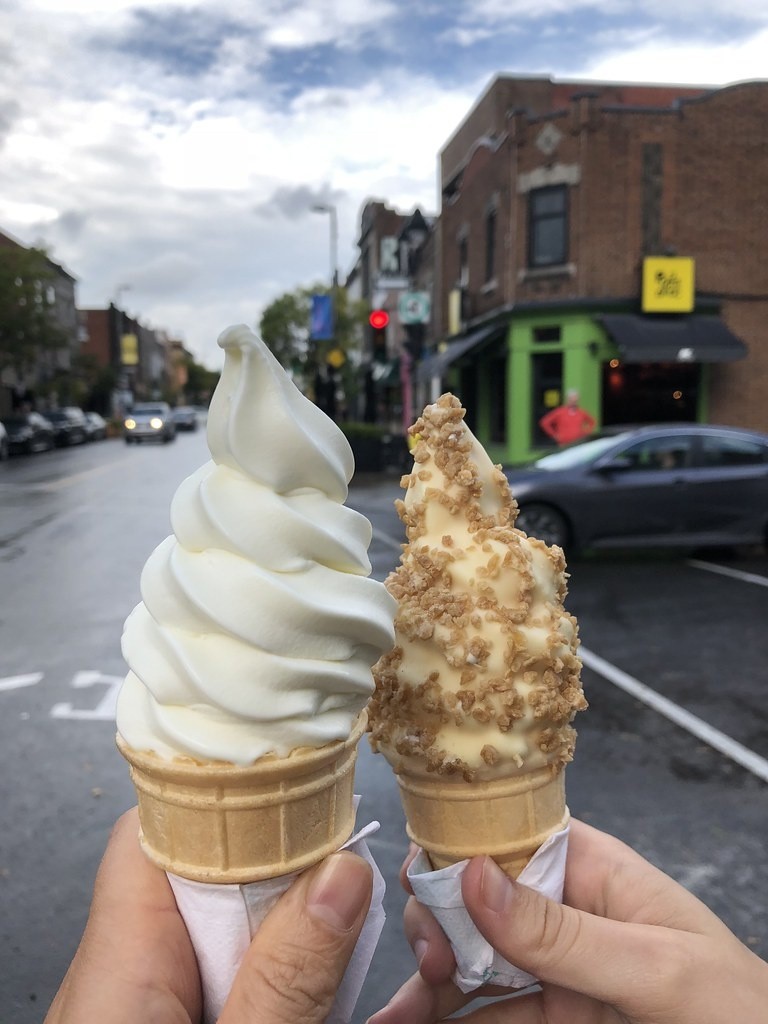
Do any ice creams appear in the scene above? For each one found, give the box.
[113,323,400,886]
[364,392,591,891]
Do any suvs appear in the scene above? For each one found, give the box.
[125,402,176,444]
[42,407,88,448]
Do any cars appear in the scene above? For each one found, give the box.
[83,412,110,442]
[499,424,768,565]
[0,423,10,462]
[171,405,198,431]
[2,413,57,455]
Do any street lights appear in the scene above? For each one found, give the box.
[312,204,337,285]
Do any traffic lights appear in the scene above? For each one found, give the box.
[402,320,428,362]
[369,310,390,367]
[312,375,338,416]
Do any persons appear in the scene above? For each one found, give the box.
[40,799,768,1023]
[539,390,597,445]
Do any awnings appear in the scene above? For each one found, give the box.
[595,317,749,364]
[409,321,505,381]
